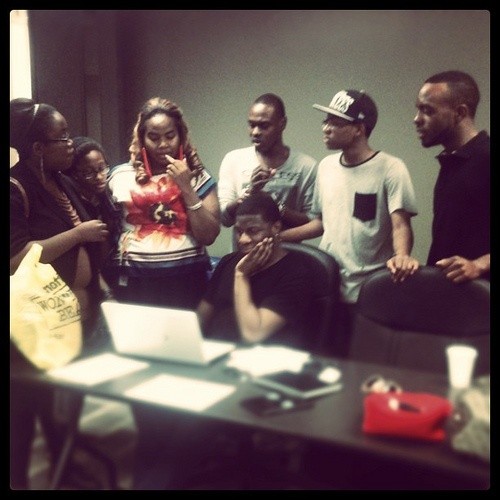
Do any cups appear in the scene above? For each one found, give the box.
[445,345,477,390]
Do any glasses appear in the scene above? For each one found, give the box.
[41,134,73,146]
[76,164,110,180]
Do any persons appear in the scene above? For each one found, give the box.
[277,87,422,357]
[104,97,222,458]
[10,96,122,490]
[217,93,318,252]
[412,70,491,283]
[195,193,328,492]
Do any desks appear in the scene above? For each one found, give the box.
[34,343,490,491]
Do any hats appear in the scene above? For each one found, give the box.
[311,90,378,134]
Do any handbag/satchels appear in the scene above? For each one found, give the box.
[10,243,81,369]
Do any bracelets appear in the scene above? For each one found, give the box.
[187,202,203,210]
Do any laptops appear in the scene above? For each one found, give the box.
[101,301,236,365]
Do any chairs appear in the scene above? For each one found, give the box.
[279,241,489,376]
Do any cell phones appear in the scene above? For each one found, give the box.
[241,394,312,417]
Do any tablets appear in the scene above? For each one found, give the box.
[257,369,343,401]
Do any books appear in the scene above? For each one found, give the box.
[253,368,343,400]
